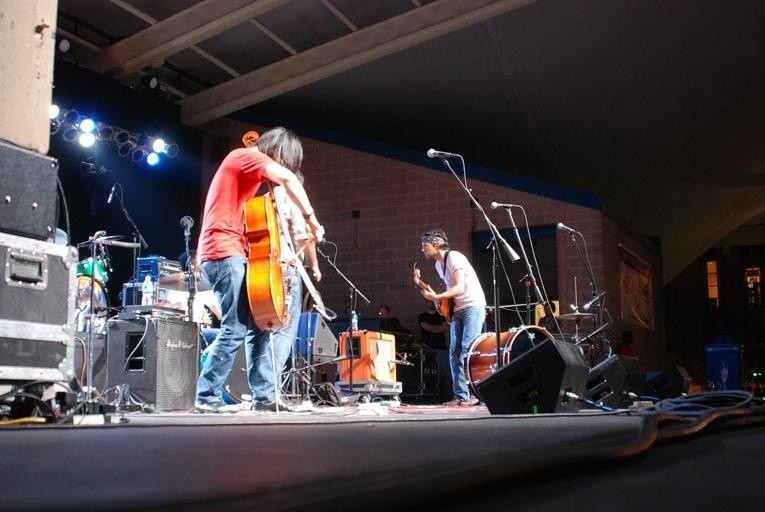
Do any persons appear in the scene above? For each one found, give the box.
[378,305,391,316]
[538,302,562,334]
[345,306,360,318]
[415,228,489,408]
[195,128,326,414]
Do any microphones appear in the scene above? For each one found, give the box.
[180,215,194,236]
[427,148,457,159]
[106,184,115,203]
[583,292,606,310]
[489,202,520,210]
[557,222,575,233]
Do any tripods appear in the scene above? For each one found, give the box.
[280,338,326,405]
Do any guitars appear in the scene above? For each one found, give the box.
[413,262,455,322]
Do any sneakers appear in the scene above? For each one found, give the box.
[192,403,240,416]
[251,399,296,413]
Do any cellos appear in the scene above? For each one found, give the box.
[242,131,289,331]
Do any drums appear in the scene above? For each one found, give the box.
[464,326,556,402]
[79,255,109,284]
[77,274,108,334]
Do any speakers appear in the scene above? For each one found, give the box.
[106,318,199,413]
[0,142,58,242]
[290,311,340,358]
[476,338,589,415]
[643,363,693,399]
[580,354,641,409]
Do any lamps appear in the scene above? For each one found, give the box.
[49,104,179,165]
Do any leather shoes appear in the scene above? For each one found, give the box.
[459,396,481,406]
[443,396,465,407]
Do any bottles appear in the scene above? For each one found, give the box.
[350,310,358,331]
[141,274,155,309]
